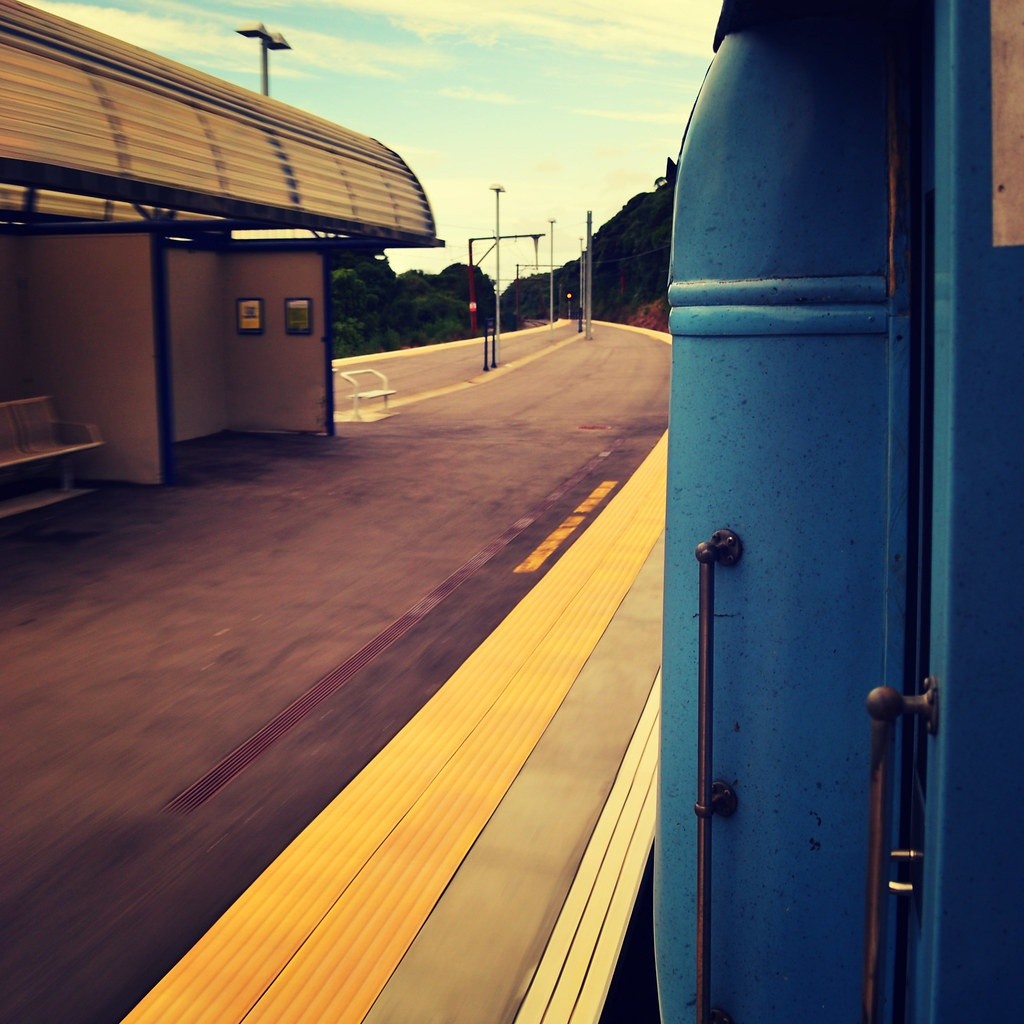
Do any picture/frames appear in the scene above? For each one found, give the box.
[236,296,265,336]
[283,296,313,337]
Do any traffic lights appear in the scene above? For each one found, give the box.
[565,292,573,299]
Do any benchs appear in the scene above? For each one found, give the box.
[1,395,107,533]
[341,369,400,424]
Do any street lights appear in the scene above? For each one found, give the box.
[236,20,294,97]
